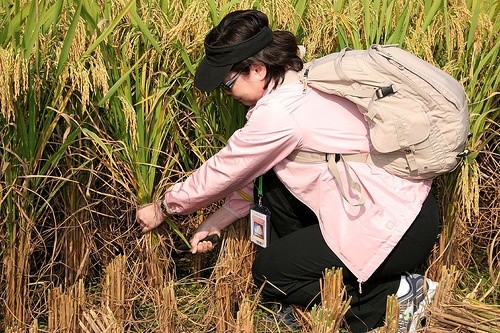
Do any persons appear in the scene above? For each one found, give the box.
[136,8,440,333]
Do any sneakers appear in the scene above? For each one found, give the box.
[396,271,439,333]
[279,305,309,330]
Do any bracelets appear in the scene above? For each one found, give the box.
[160,201,171,218]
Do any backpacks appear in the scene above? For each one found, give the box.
[270,44,469,206]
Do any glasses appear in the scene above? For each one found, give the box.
[220,69,242,91]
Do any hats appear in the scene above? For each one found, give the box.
[194,26,273,92]
